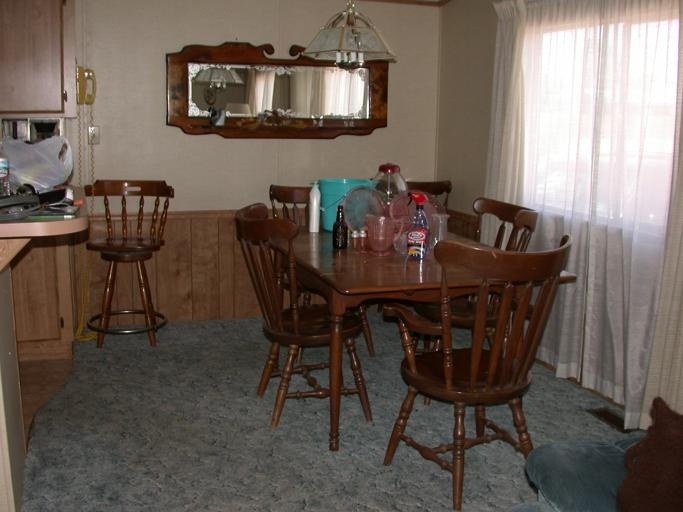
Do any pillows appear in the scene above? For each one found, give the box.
[525,437,643,512]
[618,397,682,511]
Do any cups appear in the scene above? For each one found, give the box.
[431,214,450,243]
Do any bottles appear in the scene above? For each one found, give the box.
[351,231,359,249]
[359,230,366,250]
[0,142,11,197]
[307,181,321,233]
[372,163,407,207]
[332,205,347,249]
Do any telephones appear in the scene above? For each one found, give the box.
[78,66,96,105]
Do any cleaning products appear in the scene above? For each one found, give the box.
[407,191,430,262]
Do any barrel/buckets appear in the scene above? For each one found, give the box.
[319,177,372,232]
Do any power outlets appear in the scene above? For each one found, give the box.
[88,127,100,145]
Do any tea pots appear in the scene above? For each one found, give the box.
[367,216,404,256]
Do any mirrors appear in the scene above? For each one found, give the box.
[165,41,387,138]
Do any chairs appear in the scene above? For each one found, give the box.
[270,184,326,309]
[236,202,373,424]
[372,178,450,232]
[413,197,537,405]
[383,234,572,511]
[84,181,174,347]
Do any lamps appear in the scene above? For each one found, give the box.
[191,67,244,114]
[302,0,395,65]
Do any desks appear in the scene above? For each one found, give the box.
[273,225,577,451]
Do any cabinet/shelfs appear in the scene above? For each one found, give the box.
[1,209,90,359]
